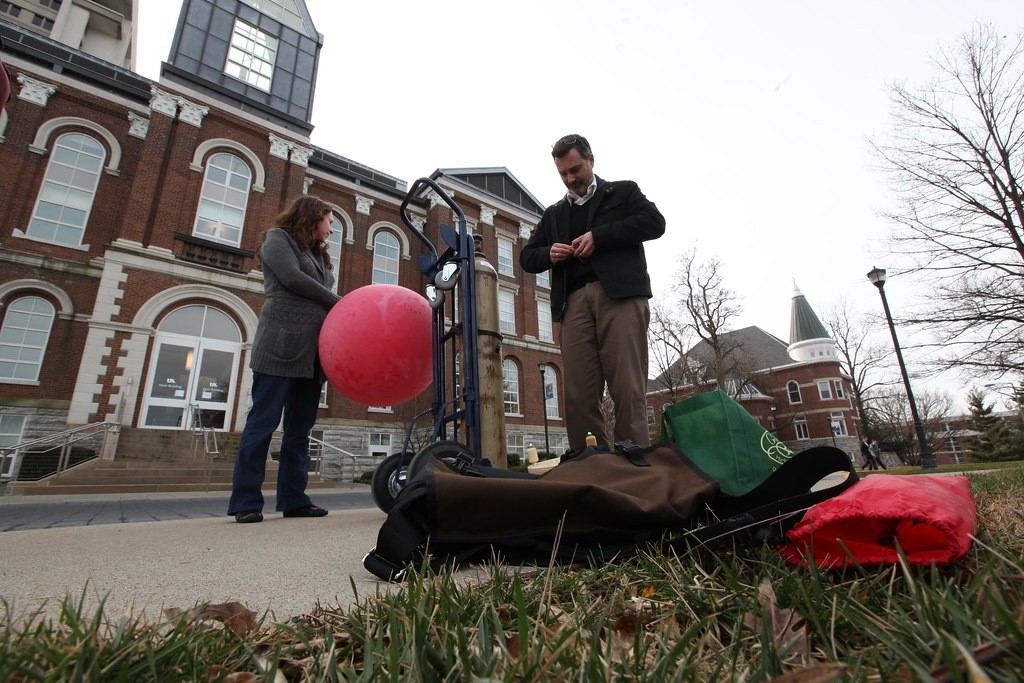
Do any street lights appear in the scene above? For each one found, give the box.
[867,265,938,468]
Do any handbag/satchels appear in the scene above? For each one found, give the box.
[364,389,979,583]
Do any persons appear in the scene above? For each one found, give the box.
[227,197,343,522]
[519,134,666,452]
[869,440,887,470]
[861,435,878,470]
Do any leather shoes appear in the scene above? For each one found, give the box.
[283,504,328,517]
[235,511,263,522]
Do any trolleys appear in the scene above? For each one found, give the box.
[362,176,490,513]
